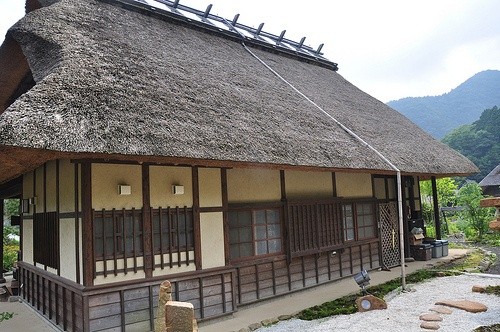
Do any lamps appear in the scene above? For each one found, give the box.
[21,199,29,215]
